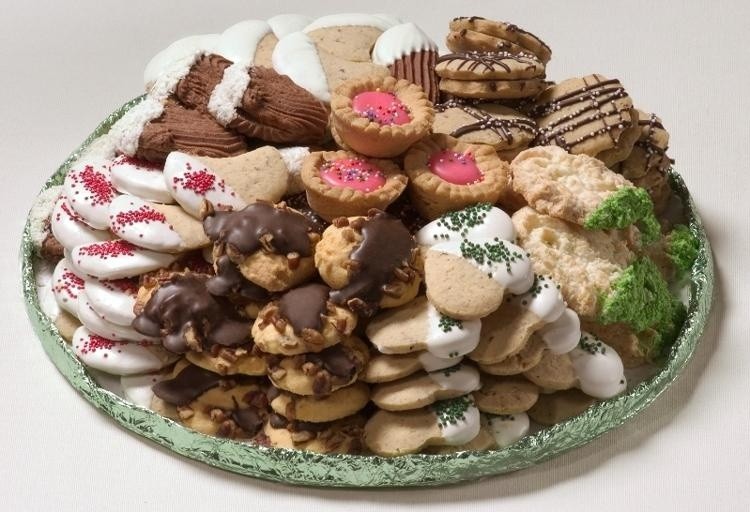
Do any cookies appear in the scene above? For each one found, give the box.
[29,12,701,456]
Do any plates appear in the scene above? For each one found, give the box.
[19,78,715,491]
